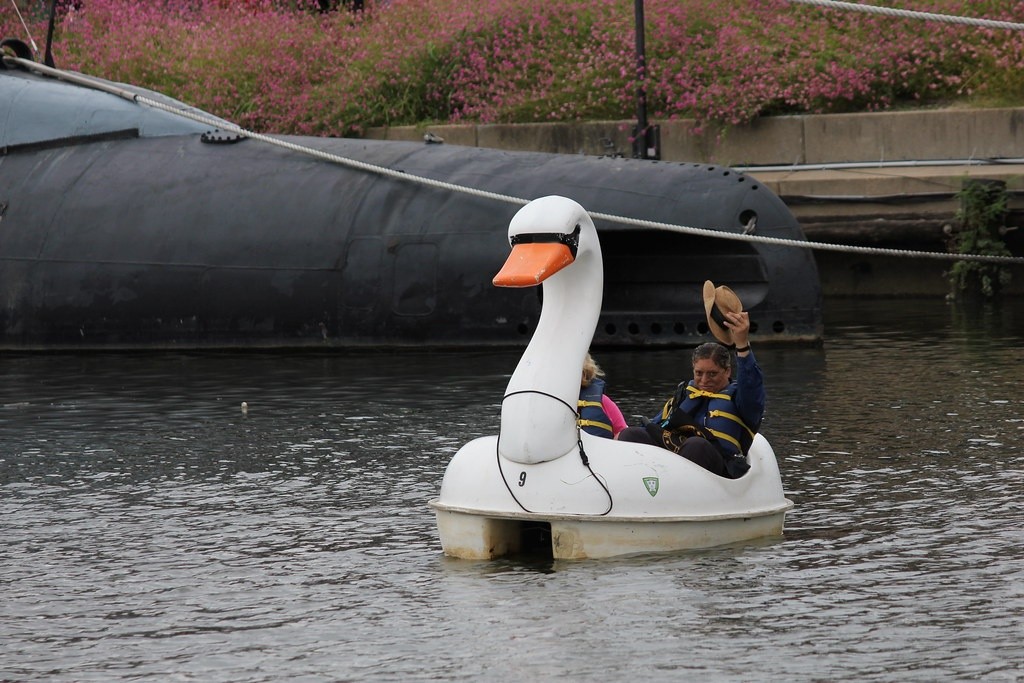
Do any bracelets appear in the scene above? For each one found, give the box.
[736,341,750,352]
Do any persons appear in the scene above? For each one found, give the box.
[577,352,628,440]
[620,312,765,479]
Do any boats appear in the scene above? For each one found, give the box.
[428,194,796,562]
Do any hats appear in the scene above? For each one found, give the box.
[703,280,742,345]
